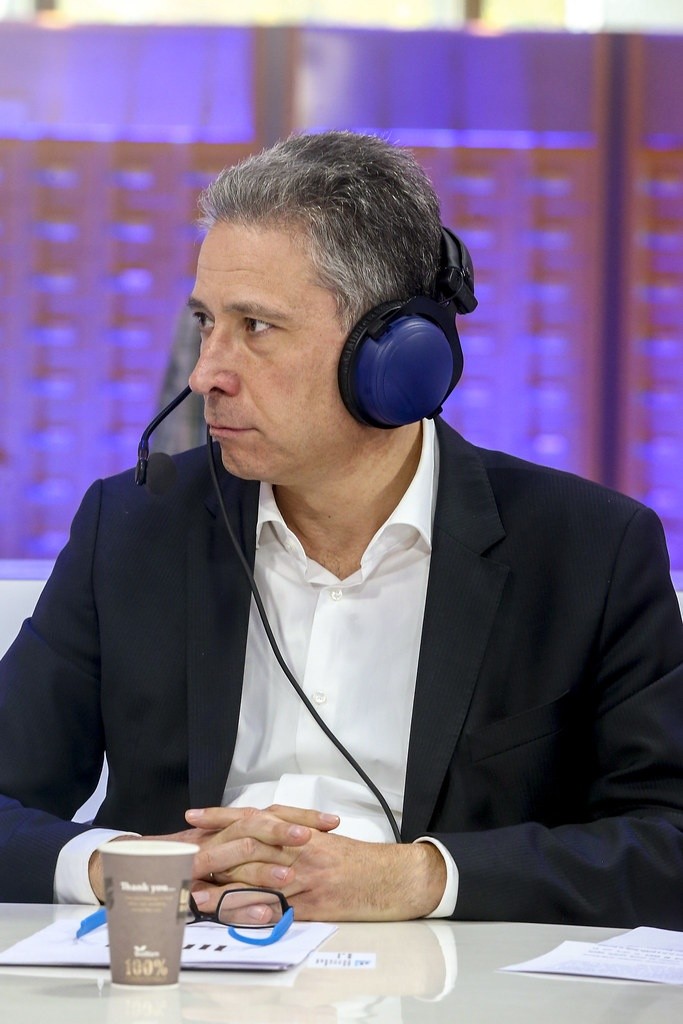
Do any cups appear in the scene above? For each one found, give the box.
[97,840,201,990]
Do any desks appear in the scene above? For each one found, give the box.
[1,900,683,1023]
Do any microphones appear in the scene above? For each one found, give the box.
[134,386,191,486]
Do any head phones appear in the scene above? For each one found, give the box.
[339,225,478,429]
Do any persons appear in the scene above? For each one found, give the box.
[0,129,683,925]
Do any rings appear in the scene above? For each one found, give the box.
[208,872,216,886]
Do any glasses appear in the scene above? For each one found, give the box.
[76,888,294,947]
